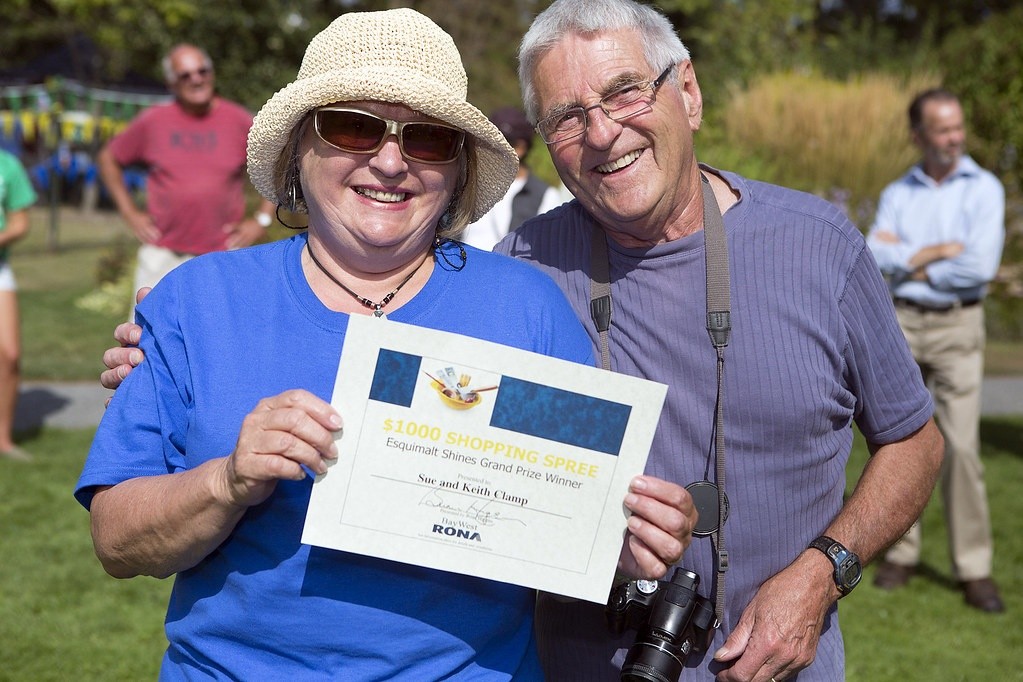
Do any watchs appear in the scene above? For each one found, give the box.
[254,210,272,228]
[808,536,863,600]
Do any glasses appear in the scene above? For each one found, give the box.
[313,107,466,166]
[172,66,212,83]
[534,62,676,144]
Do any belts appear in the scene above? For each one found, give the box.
[894,297,979,313]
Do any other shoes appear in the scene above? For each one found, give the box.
[874,561,918,588]
[954,578,1003,613]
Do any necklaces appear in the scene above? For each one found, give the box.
[306,236,467,321]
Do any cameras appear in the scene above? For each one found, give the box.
[600,566,719,682]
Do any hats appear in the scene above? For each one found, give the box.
[247,8,519,227]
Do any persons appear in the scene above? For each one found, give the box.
[74,6,699,682]
[99,1,945,682]
[863,88,1005,613]
[0,146,39,463]
[97,44,278,325]
